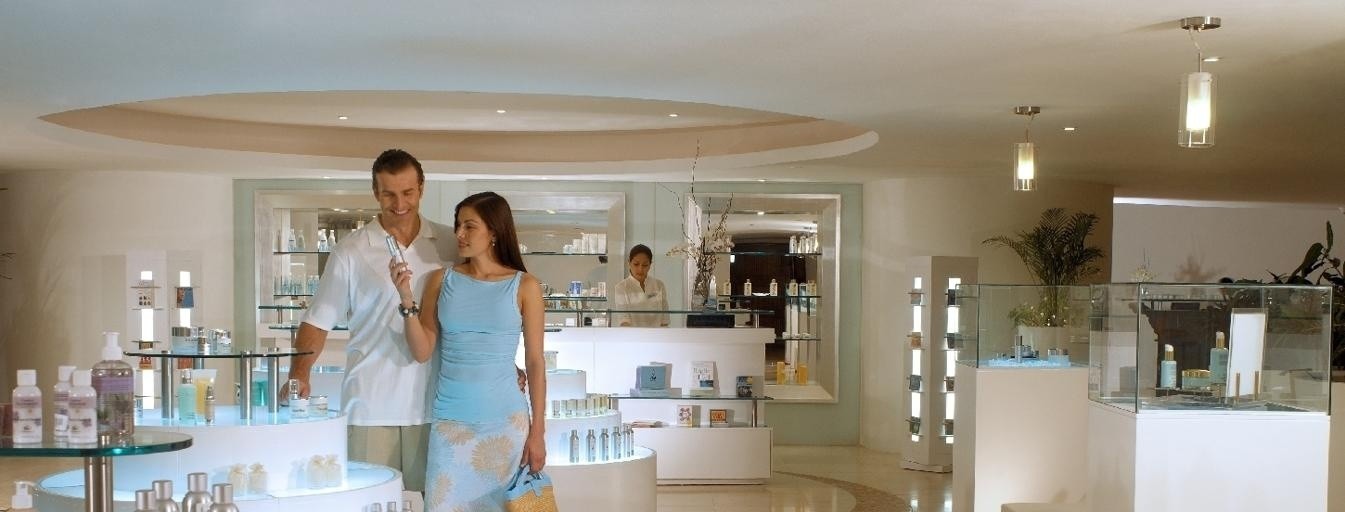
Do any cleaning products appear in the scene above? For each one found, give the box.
[569,422,634,463]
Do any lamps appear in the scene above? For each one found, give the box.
[1177,17,1221,149]
[1012,106,1040,192]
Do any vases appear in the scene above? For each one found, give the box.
[691,270,711,312]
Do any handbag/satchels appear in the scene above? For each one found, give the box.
[502,463,558,511]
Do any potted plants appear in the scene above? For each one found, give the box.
[982,208,1105,359]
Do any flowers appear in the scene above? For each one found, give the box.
[659,137,734,302]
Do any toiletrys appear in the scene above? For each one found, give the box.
[518,359,821,464]
[1181,368,1212,390]
[537,232,610,327]
[1159,343,1177,388]
[721,232,819,296]
[1210,331,1229,385]
[995,334,1071,367]
[10,219,412,512]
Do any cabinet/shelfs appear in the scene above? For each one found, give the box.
[683,192,841,404]
[900,256,978,472]
[514,308,775,486]
[521,350,657,512]
[33,346,404,511]
[232,179,627,412]
[1,424,193,512]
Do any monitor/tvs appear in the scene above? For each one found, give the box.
[684,314,735,328]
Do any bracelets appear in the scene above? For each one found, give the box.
[398,301,419,318]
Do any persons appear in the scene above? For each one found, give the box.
[388,192,548,512]
[614,243,671,328]
[277,148,528,495]
